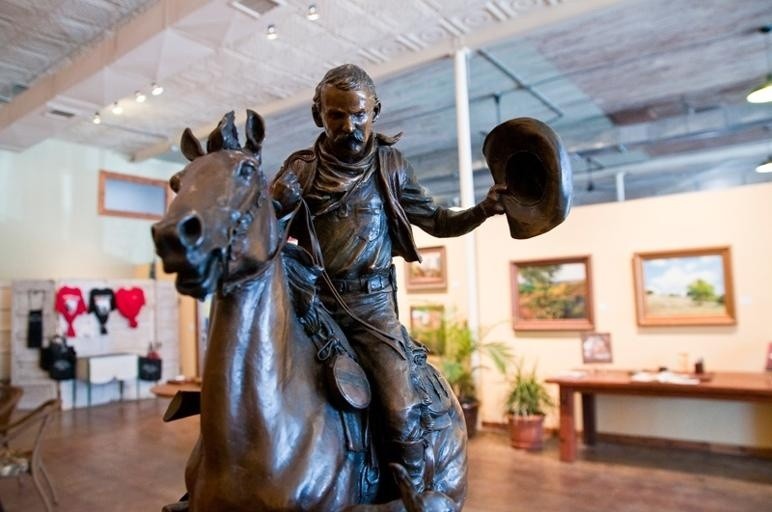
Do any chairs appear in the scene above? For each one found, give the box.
[0,383,25,488]
[0,398,60,512]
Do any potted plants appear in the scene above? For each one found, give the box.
[505,357,556,449]
[404,320,515,437]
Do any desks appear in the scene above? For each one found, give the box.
[72,352,140,416]
[545,368,772,463]
[150,381,202,398]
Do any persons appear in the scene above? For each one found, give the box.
[267,62,510,512]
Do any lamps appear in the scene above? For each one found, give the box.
[92,82,166,126]
[753,152,771,176]
[745,73,772,106]
[265,3,320,40]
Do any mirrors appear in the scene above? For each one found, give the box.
[96,168,175,221]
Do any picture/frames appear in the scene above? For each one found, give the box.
[403,246,448,293]
[579,332,615,364]
[409,305,447,355]
[506,254,596,331]
[630,245,737,328]
